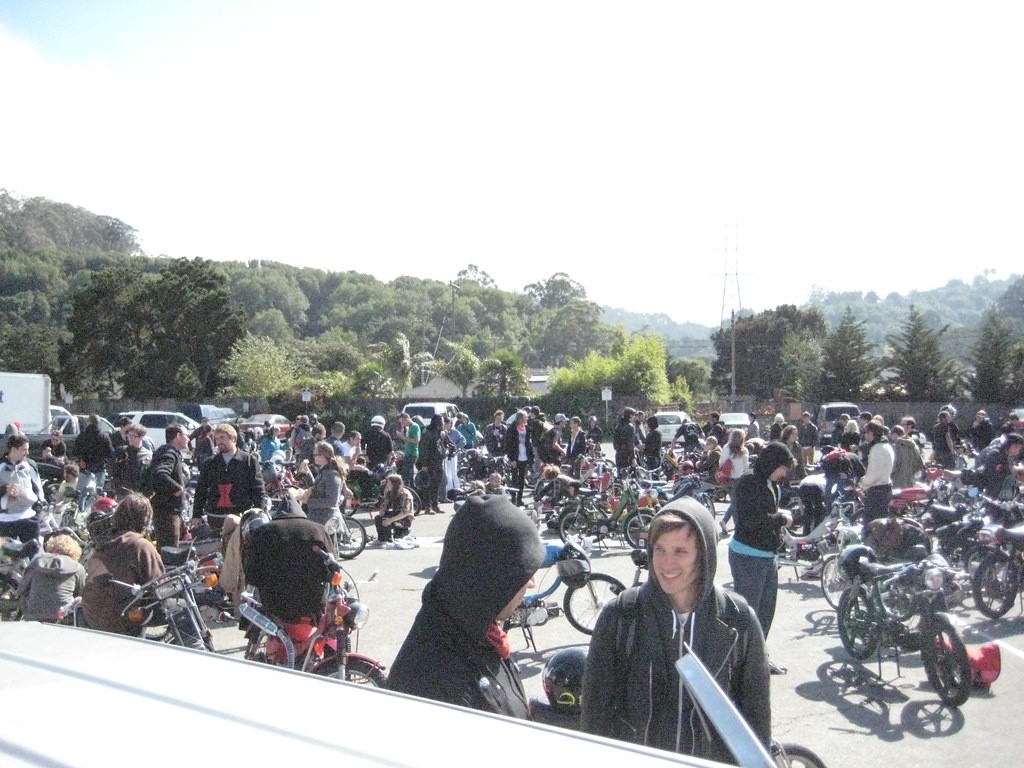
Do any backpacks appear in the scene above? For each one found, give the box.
[537,428,560,463]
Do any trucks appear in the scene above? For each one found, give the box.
[0,371,73,436]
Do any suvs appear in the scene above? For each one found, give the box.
[112,410,203,463]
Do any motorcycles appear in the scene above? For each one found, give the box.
[0,445,1024,768]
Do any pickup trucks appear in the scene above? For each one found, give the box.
[22,414,123,453]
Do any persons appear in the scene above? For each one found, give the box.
[0,402,1024,676]
[579,495,772,768]
[382,492,549,719]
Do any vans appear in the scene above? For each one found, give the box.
[401,401,461,433]
[815,401,862,445]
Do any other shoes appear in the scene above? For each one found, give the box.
[720,520,728,534]
[374,540,391,546]
[768,660,787,675]
[432,507,445,513]
[425,508,437,514]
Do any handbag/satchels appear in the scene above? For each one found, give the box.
[714,450,734,484]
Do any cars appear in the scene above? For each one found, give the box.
[653,410,695,446]
[718,412,760,441]
[237,413,295,443]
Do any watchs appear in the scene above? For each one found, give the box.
[781,515,787,525]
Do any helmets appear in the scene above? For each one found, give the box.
[415,470,431,490]
[371,415,386,429]
[557,545,592,587]
[543,646,588,713]
[588,415,597,422]
[241,510,271,539]
[91,498,118,513]
[310,414,317,420]
[837,544,876,584]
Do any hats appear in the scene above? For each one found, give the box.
[977,410,985,417]
[554,413,570,423]
[857,412,872,420]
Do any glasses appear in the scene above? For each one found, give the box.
[901,423,909,426]
[127,433,140,438]
[312,454,322,457]
